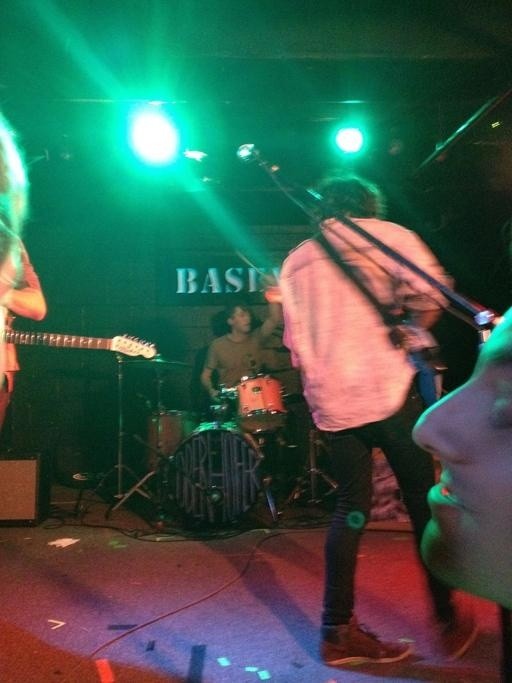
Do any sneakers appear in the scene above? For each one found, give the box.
[319,614,413,666]
[435,613,480,664]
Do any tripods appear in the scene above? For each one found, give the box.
[72,355,176,519]
[262,403,337,523]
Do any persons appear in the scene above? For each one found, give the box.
[278,178,482,668]
[199,300,283,401]
[1,218,47,426]
[409,308,512,615]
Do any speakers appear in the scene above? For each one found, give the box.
[0,447,54,527]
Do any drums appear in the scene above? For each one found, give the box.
[164,422,265,522]
[234,372,289,434]
[147,409,206,459]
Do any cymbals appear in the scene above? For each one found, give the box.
[123,354,192,369]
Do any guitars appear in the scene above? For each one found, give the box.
[265,286,439,364]
[1,330,157,358]
